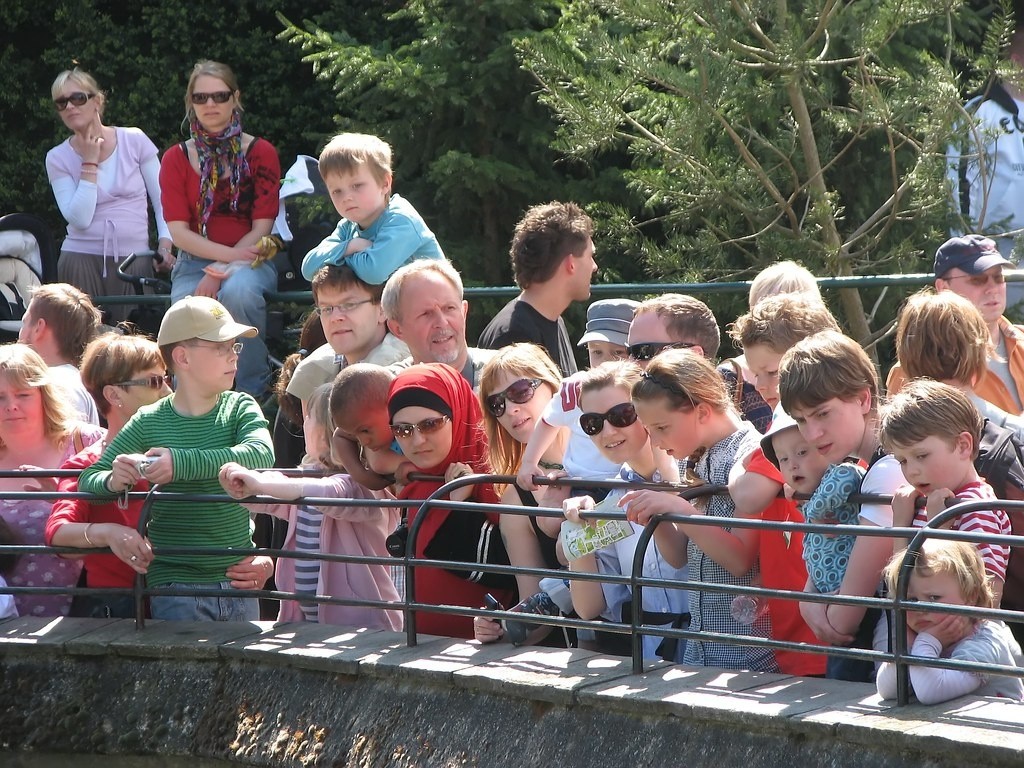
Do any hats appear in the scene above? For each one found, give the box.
[759,400,799,472]
[157,295,258,357]
[934,234,1017,279]
[577,299,645,350]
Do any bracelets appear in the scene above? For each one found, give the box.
[538,461,564,470]
[84,522,94,546]
[204,265,228,279]
[157,248,171,253]
[81,162,97,174]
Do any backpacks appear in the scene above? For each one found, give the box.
[284,200,342,290]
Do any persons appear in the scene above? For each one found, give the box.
[883,378,1012,609]
[159,60,281,402]
[78,294,276,624]
[946,0,1024,309]
[44,333,173,589]
[18,282,100,428]
[301,132,446,285]
[219,262,891,678]
[0,345,109,615]
[46,70,176,325]
[896,286,1024,445]
[476,201,598,378]
[870,537,1024,705]
[887,233,1024,419]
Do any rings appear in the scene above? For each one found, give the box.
[255,581,258,586]
[131,555,136,561]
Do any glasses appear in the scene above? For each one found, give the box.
[389,415,450,438]
[623,341,698,361]
[942,269,1008,287]
[111,374,173,389]
[315,297,373,318]
[191,90,235,104]
[580,402,637,436]
[484,379,543,418]
[179,341,245,358]
[54,92,96,111]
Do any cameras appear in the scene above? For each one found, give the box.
[128,454,162,480]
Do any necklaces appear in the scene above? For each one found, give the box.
[100,435,110,448]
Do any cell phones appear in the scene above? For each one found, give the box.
[485,593,504,640]
